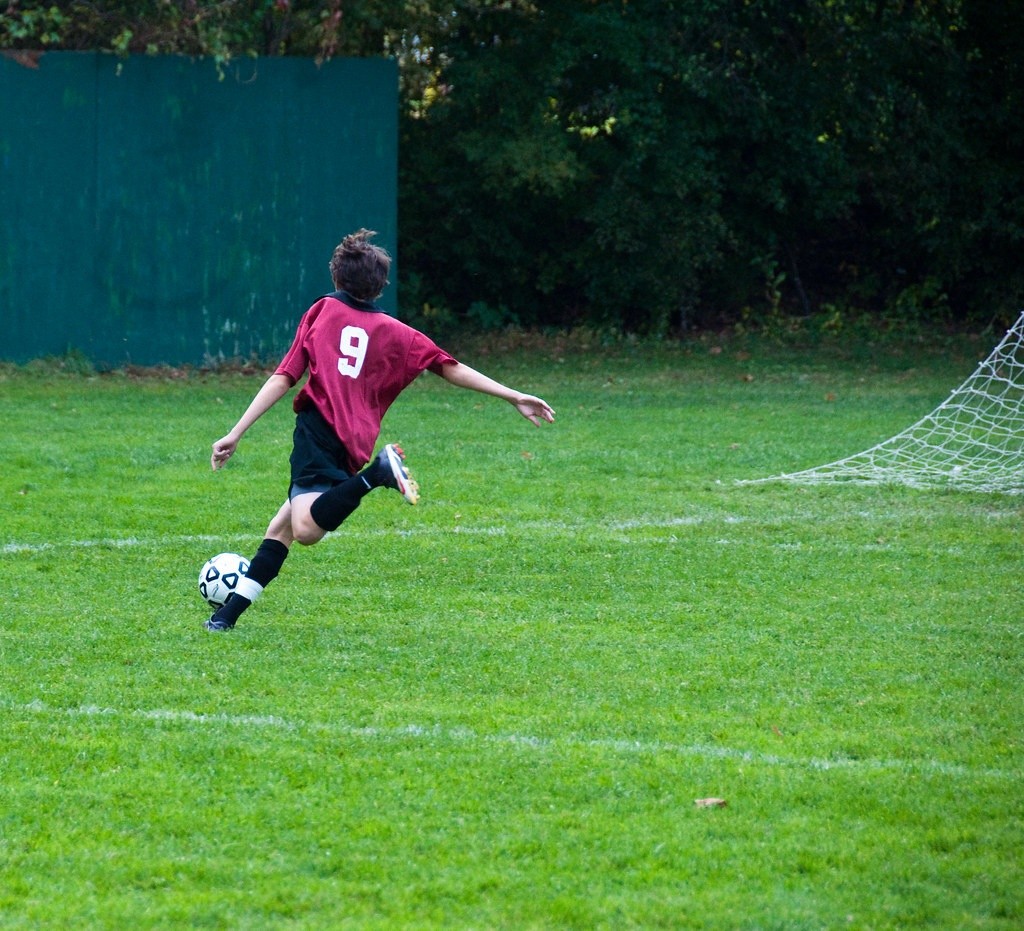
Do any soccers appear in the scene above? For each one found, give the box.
[197,551,251,611]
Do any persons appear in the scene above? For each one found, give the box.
[202,228,556,633]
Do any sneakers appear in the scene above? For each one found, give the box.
[201,613,234,633]
[376,443,420,505]
